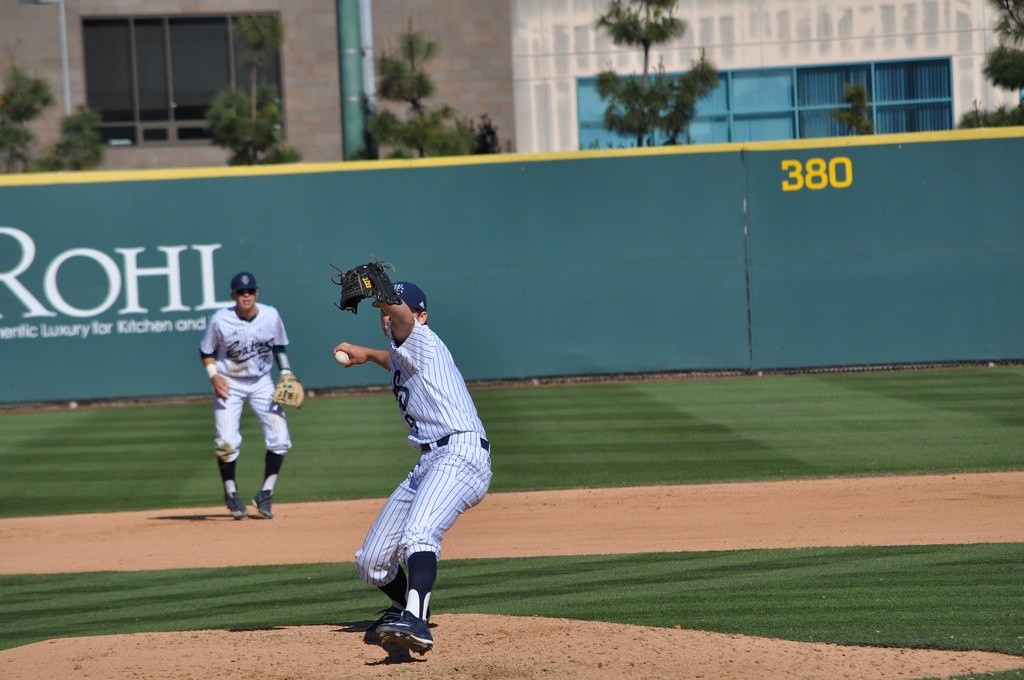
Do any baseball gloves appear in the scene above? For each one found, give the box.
[273,373,305,409]
[340,262,403,316]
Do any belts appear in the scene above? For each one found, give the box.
[421,435,489,453]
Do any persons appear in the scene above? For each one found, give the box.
[332,263,491,655]
[199,272,304,519]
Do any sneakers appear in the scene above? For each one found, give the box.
[223,490,247,518]
[252,489,273,518]
[368,606,402,630]
[376,610,434,656]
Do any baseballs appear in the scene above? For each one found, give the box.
[334,350,349,366]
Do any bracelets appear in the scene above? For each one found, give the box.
[206,364,216,379]
[280,370,291,376]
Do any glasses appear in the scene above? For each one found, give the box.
[236,289,256,295]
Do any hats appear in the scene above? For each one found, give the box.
[372,282,428,311]
[231,272,259,290]
